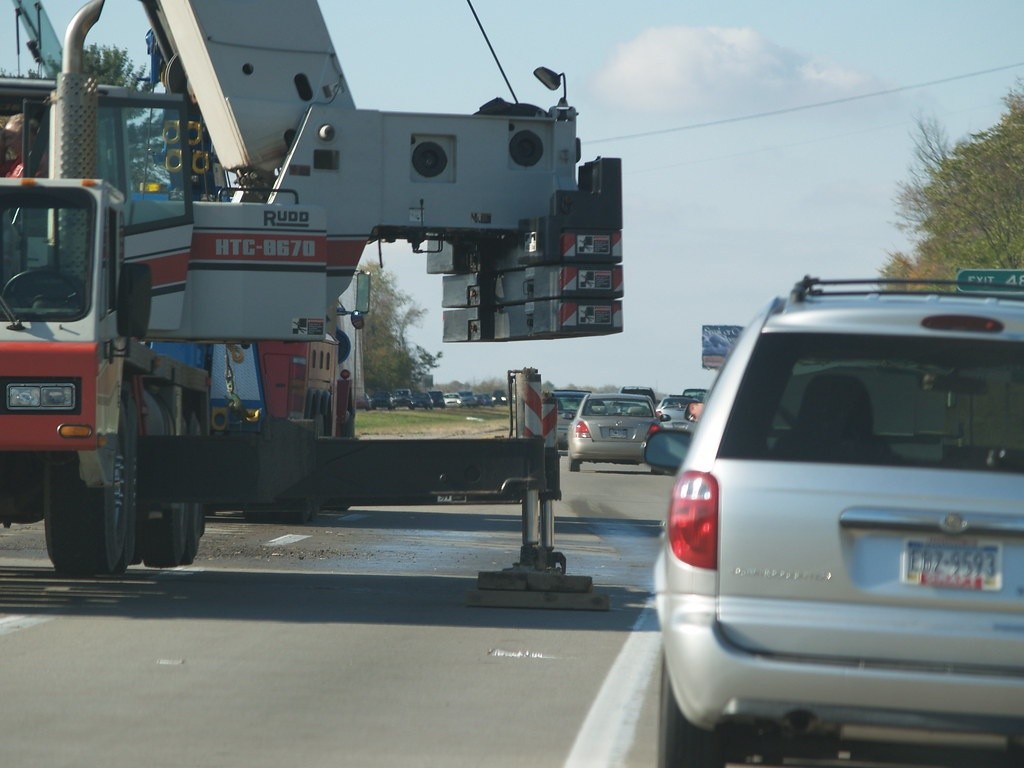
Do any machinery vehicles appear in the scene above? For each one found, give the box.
[0,0,629,579]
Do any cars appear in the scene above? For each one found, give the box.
[560,392,671,472]
[356,389,516,412]
[552,388,606,450]
[655,388,709,432]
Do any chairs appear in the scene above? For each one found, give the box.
[773,372,895,465]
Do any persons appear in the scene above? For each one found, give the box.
[2,113,48,179]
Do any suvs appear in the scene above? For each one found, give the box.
[619,386,657,406]
[642,272,1024,768]
